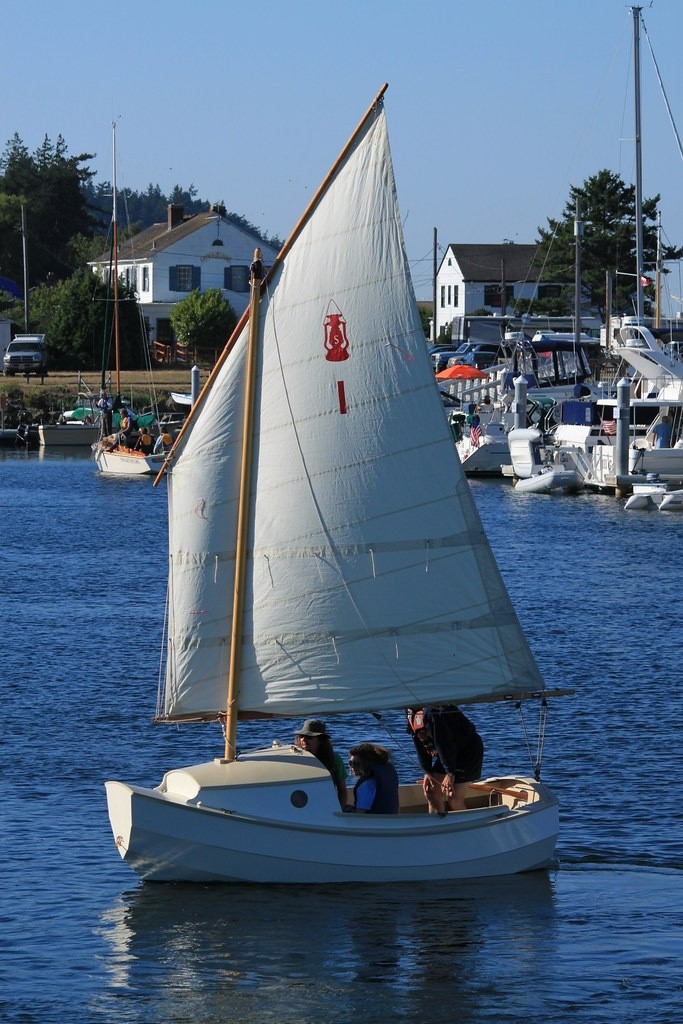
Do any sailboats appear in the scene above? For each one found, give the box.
[450,4,683,508]
[102,82,562,890]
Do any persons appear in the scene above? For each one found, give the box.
[406,705,484,813]
[479,395,493,411]
[466,402,477,437]
[652,416,673,448]
[349,743,400,814]
[2,380,178,455]
[293,719,348,815]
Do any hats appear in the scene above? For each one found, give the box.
[100,391,106,398]
[294,719,331,739]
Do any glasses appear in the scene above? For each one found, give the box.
[348,760,362,767]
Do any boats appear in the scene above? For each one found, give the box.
[90,439,171,476]
[38,396,121,445]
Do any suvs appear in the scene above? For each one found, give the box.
[431,343,478,370]
[4,335,48,375]
[448,344,512,371]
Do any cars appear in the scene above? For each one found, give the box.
[427,343,458,353]
[463,352,503,371]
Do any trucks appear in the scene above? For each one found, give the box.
[532,330,601,357]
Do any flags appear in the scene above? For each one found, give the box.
[470,415,481,446]
[601,419,616,434]
